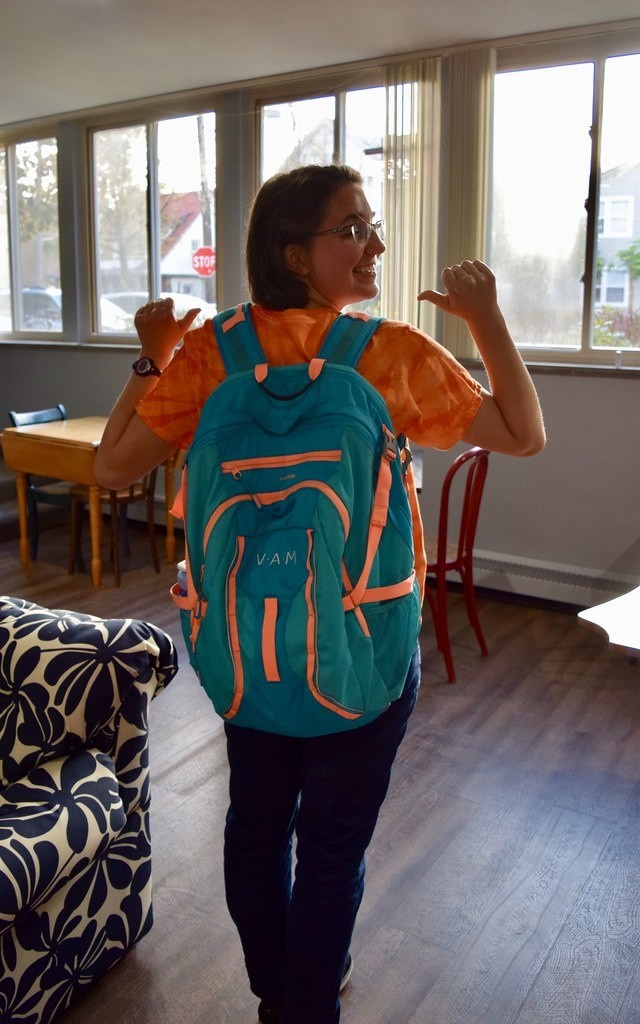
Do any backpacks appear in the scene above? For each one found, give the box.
[169,302,422,735]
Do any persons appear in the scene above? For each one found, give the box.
[92,161,546,1024]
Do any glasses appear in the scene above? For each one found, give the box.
[314,220,386,246]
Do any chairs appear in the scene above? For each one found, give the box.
[421,446,491,682]
[11,405,131,572]
[68,462,162,576]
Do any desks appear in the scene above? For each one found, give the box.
[2,415,176,587]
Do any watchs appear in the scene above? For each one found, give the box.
[132,357,163,377]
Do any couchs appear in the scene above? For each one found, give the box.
[0,592,178,1023]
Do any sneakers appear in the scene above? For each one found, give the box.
[339,951,353,992]
